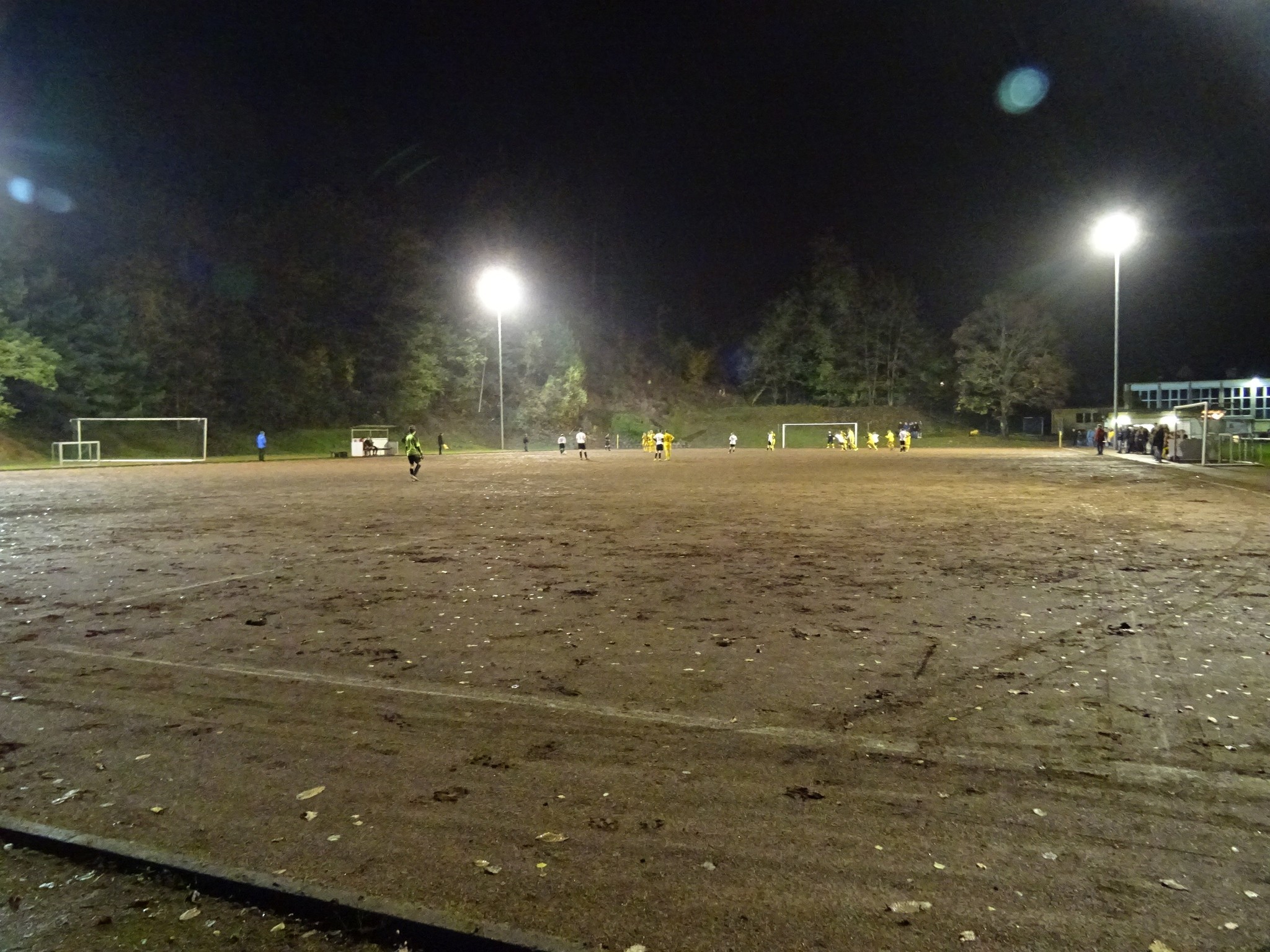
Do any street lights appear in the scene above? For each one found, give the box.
[1087,209,1145,450]
[475,267,524,450]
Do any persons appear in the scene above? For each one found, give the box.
[826,420,923,454]
[1070,423,1169,463]
[558,434,566,455]
[523,434,529,452]
[642,429,656,452]
[438,433,444,454]
[604,434,611,451]
[663,430,675,461]
[576,429,589,460]
[766,431,775,451]
[652,429,664,461]
[256,431,266,461]
[405,425,424,482]
[728,432,737,454]
[363,436,378,456]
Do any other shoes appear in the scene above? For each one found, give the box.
[653,458,656,461]
[664,459,670,461]
[411,474,419,482]
[658,459,661,461]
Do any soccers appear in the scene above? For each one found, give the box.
[647,380,652,384]
